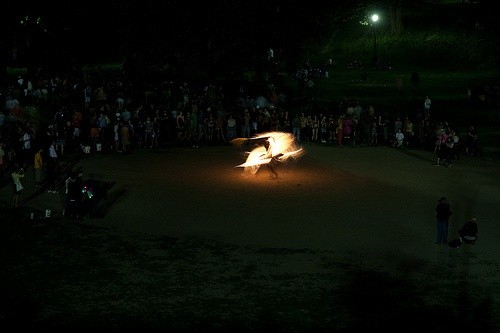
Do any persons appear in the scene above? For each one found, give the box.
[74,172,83,187]
[83,173,99,213]
[49,140,59,177]
[62,175,74,215]
[1,48,499,167]
[434,197,450,247]
[11,166,24,209]
[34,148,45,186]
[45,156,58,194]
[456,216,479,249]
[70,183,80,218]
